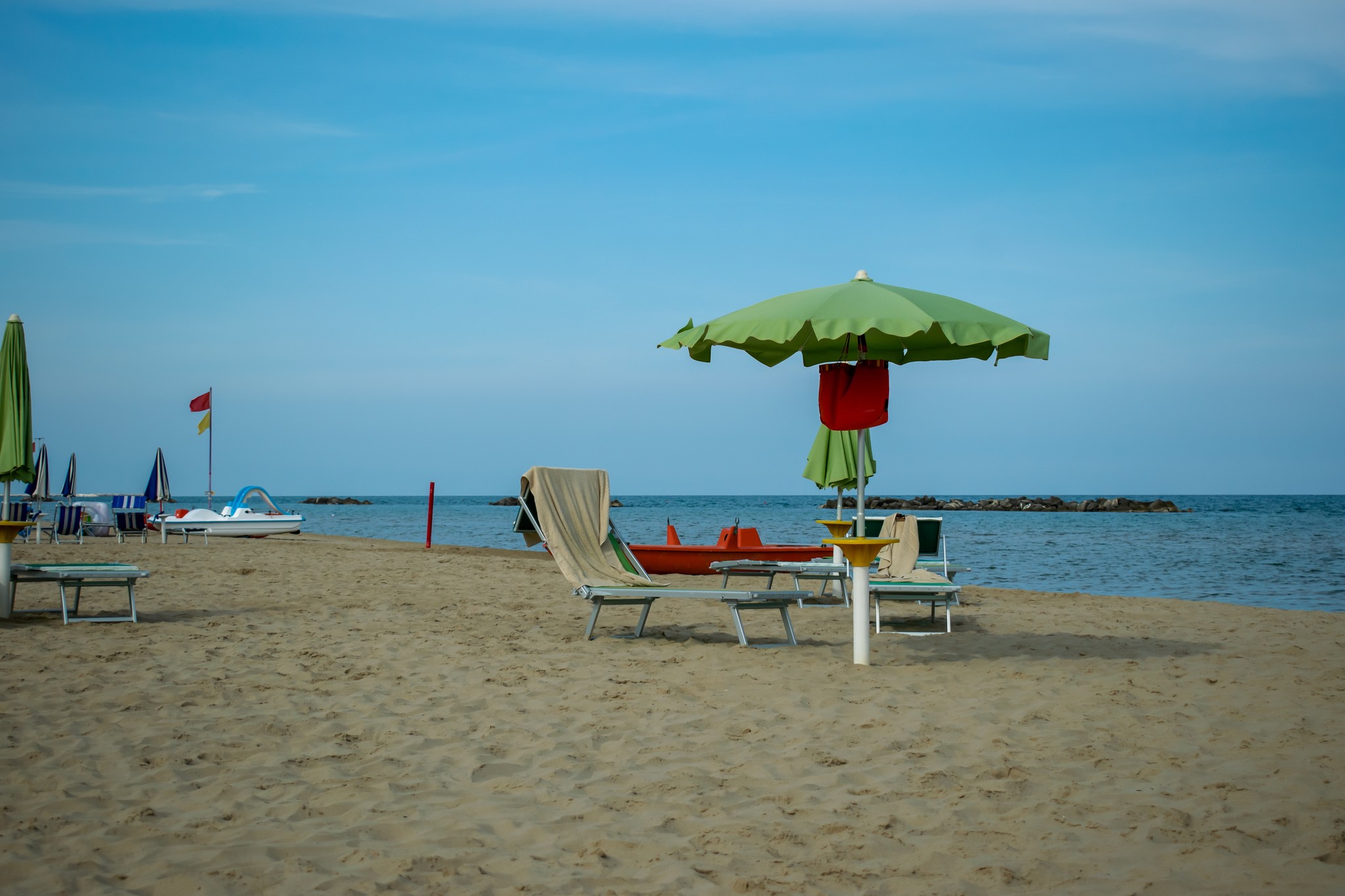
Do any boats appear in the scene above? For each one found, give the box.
[140,487,306,540]
[543,508,844,575]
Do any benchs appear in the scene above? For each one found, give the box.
[12,563,150,626]
[110,495,149,546]
[709,560,850,610]
[164,527,211,544]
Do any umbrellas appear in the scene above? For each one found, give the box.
[655,267,1050,668]
[23,443,50,545]
[0,314,37,523]
[60,452,76,506]
[144,447,171,540]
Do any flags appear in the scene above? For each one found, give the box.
[189,391,212,412]
[196,410,212,436]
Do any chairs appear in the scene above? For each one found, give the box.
[852,514,960,641]
[510,465,812,649]
[0,500,33,543]
[51,503,87,546]
